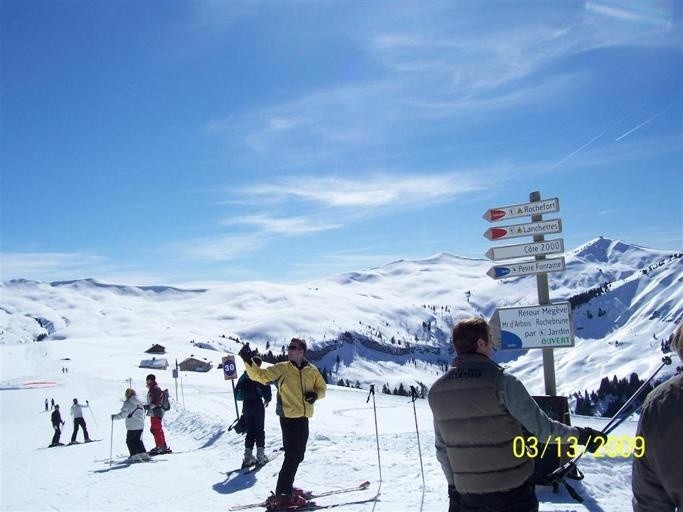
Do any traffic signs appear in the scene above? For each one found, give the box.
[496,301,575,352]
[483,198,566,280]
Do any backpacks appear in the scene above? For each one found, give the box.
[160,389,170,411]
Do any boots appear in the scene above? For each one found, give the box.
[244,448,257,466]
[257,447,271,464]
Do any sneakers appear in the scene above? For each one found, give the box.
[128,446,167,460]
[291,488,303,495]
[275,494,305,506]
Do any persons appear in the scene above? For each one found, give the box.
[427,317,608,512]
[236,336,327,506]
[631,321,682,511]
[69,397,94,443]
[141,373,166,454]
[110,388,147,456]
[50,397,54,410]
[44,398,48,410]
[49,403,63,445]
[232,354,272,466]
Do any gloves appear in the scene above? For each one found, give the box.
[238,342,252,365]
[447,484,461,512]
[575,427,608,453]
[304,391,318,404]
[143,405,149,410]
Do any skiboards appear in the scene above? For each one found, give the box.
[227,448,285,475]
[65,439,102,447]
[230,481,380,511]
[103,459,169,465]
